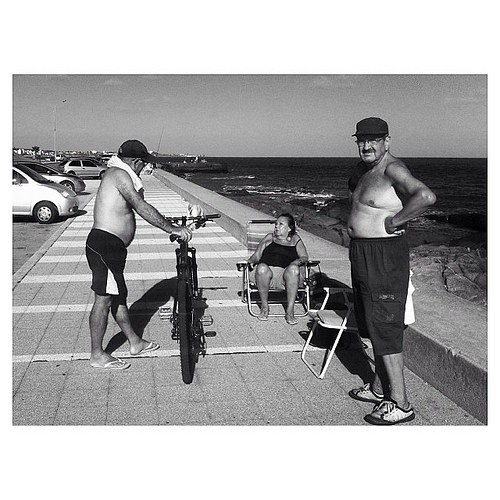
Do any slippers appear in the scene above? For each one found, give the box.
[93,356,131,370]
[133,341,160,353]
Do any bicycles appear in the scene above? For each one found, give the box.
[164,213,222,384]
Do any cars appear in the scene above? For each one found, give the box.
[37,153,114,181]
[13,157,86,195]
[12,163,80,224]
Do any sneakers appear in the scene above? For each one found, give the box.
[363,396,415,425]
[349,382,387,404]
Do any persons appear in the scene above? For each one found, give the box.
[247,212,309,324]
[86,140,192,370]
[345,117,436,425]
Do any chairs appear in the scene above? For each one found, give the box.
[234,219,322,317]
[300,270,417,379]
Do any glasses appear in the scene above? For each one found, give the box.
[355,136,386,148]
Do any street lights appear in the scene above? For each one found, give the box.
[53,99,67,163]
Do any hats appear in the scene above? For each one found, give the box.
[352,117,389,137]
[118,140,155,162]
